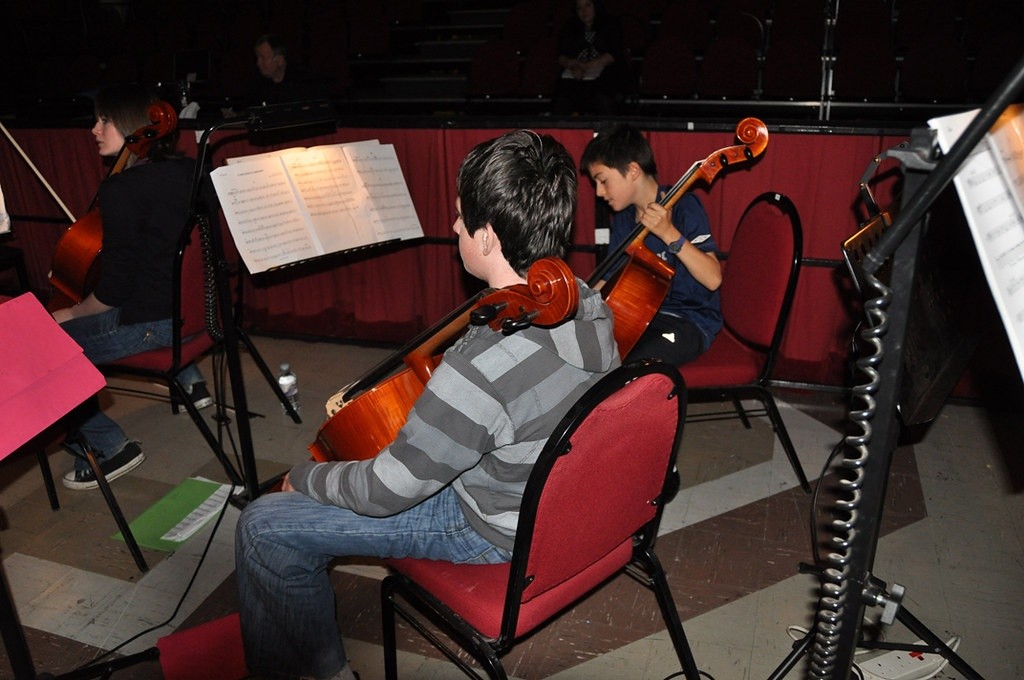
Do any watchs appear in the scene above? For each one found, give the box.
[667,236,685,253]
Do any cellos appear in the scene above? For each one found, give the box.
[38,101,178,314]
[251,252,578,506]
[587,117,769,363]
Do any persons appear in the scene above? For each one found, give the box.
[221,32,320,119]
[51,93,214,491]
[578,124,723,502]
[231,130,626,680]
[553,0,623,115]
[94,0,130,72]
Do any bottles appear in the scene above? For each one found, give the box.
[277,363,301,416]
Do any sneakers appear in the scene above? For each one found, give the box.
[177,382,211,411]
[60,438,145,490]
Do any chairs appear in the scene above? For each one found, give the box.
[378,352,702,680]
[0,0,434,123]
[95,197,303,489]
[464,0,1024,120]
[676,191,812,495]
